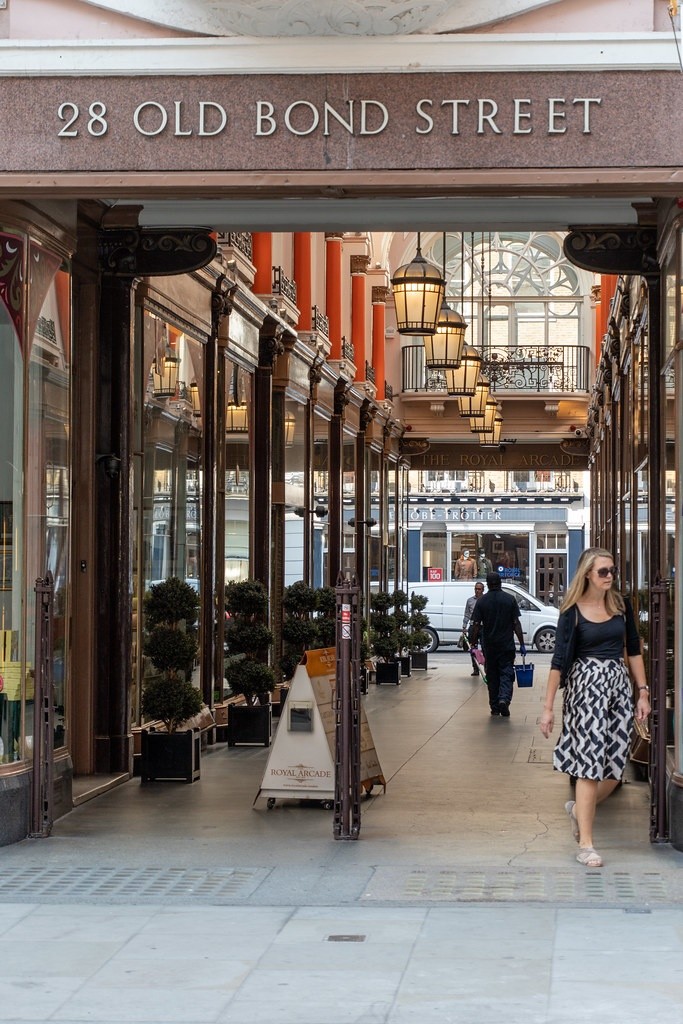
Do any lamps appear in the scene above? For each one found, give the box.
[151,321,297,448]
[390,231,504,448]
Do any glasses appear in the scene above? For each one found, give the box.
[595,567,616,576]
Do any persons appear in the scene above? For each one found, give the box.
[541,548,651,868]
[454,547,477,580]
[462,582,484,675]
[471,572,526,716]
[476,548,493,578]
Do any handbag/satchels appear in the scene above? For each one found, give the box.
[629,716,650,764]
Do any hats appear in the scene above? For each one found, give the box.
[486,572,501,585]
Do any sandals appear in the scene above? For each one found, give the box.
[565,800,580,843]
[576,847,603,867]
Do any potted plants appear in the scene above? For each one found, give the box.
[139,574,206,784]
[279,580,431,717]
[222,577,278,747]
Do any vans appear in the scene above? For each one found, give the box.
[408,580,561,653]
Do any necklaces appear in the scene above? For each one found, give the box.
[598,606,600,609]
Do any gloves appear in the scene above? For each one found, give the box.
[519,643,526,656]
[469,647,478,656]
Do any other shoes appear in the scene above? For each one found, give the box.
[491,709,498,715]
[471,671,479,676]
[499,700,510,716]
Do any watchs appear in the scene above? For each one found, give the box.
[637,686,649,691]
